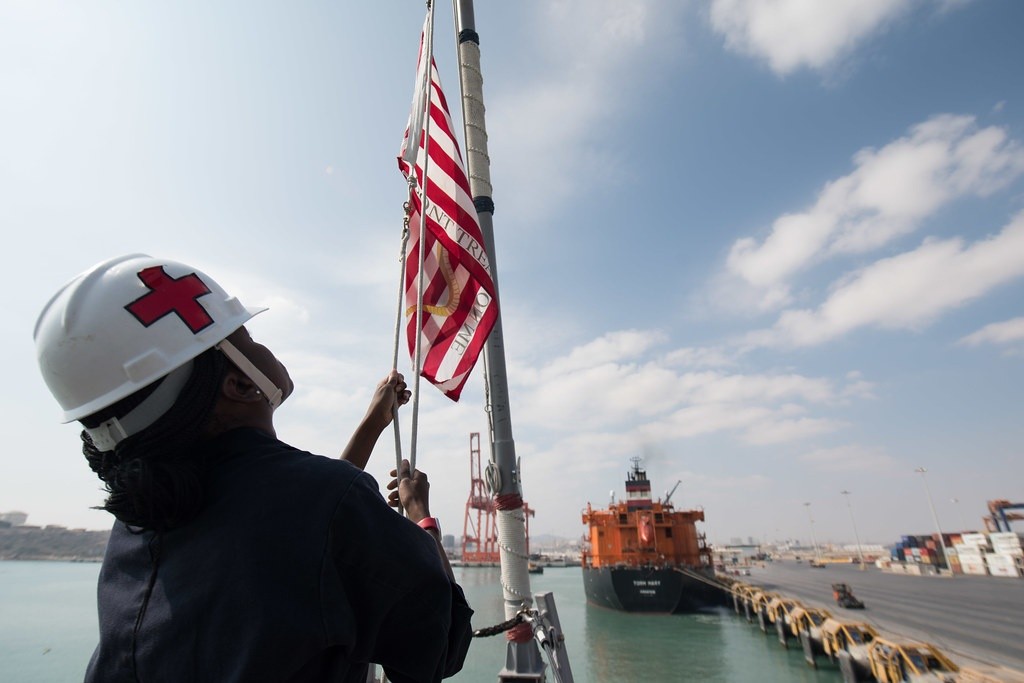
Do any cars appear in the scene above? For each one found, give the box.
[743,569,751,576]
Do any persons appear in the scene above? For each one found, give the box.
[33,253,474,683]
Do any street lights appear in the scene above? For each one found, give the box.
[915,467,955,576]
[841,491,865,564]
[804,503,821,560]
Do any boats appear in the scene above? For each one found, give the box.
[580,457,714,615]
[528,554,544,574]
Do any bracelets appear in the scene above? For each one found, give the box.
[417,517,442,543]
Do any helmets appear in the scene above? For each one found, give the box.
[31,252,271,426]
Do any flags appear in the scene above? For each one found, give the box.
[397,13,500,403]
